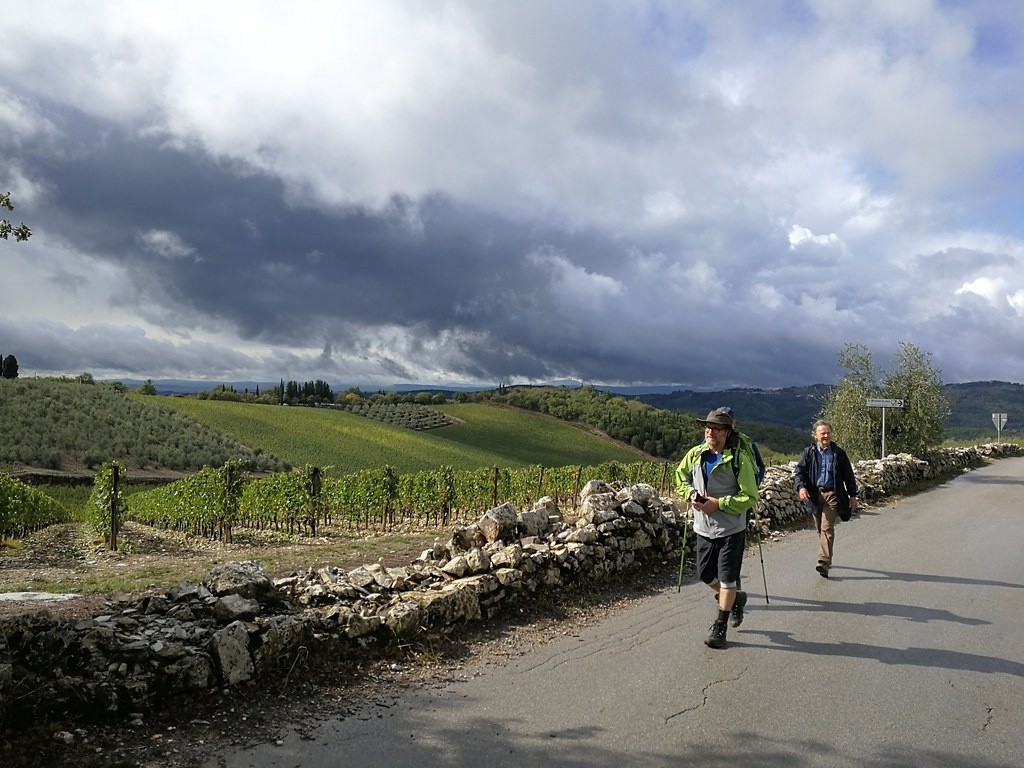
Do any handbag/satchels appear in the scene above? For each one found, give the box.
[837,487,851,521]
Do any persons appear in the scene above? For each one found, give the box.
[675,407,760,651]
[794,419,858,579]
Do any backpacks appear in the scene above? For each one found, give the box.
[693,432,765,485]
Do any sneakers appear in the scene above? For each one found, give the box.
[704,619,728,646]
[729,592,747,627]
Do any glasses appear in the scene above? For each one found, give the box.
[704,426,726,434]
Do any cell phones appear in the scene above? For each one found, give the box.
[694,492,706,504]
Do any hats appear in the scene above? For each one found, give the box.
[716,407,736,420]
[696,410,733,429]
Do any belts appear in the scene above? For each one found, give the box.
[817,487,834,492]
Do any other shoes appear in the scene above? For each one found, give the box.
[815,562,829,577]
